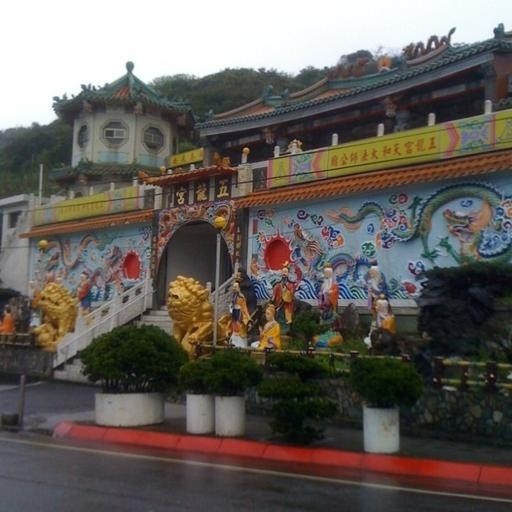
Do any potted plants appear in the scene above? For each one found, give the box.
[78,321,262,439]
[346,355,425,457]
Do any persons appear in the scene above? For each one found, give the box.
[227,257,401,355]
[0,304,18,350]
[75,270,91,314]
